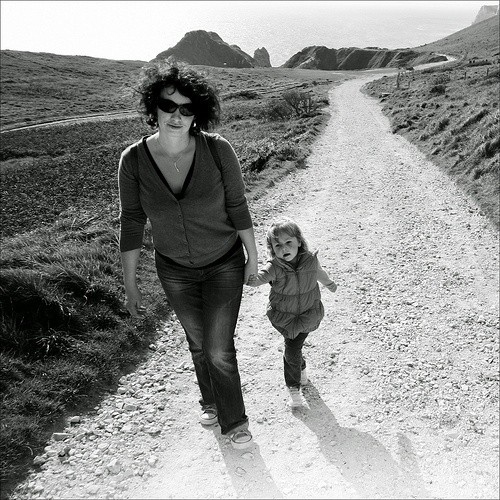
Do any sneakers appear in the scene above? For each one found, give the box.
[288,387,302,409]
[200,407,218,425]
[230,429,252,449]
[300,369,308,388]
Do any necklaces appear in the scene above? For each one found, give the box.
[155,137,191,166]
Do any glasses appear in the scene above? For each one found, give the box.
[157,98,194,116]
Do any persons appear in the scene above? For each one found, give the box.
[117,55,259,452]
[248,217,340,409]
